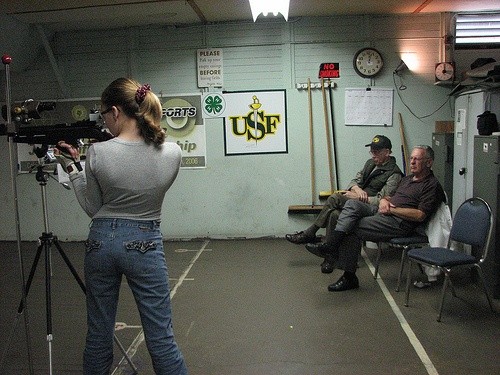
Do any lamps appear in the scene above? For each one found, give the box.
[393,61,409,75]
[250,0,290,23]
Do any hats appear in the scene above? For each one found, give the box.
[365,134,392,149]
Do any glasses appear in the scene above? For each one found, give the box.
[410,157,432,160]
[369,150,388,156]
[98,106,121,120]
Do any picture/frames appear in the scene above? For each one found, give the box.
[222,89,288,156]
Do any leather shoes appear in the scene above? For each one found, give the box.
[305,243,328,258]
[320,260,333,273]
[327,276,359,292]
[286,230,316,245]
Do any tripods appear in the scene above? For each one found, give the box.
[0,148,139,375]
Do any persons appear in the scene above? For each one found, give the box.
[286,135,405,273]
[304,145,442,292]
[54,77,187,375]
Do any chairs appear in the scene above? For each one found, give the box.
[403,197,494,322]
[373,190,449,293]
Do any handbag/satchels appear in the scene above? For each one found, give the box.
[476,110,500,136]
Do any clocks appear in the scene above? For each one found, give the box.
[353,48,384,78]
[435,61,456,82]
